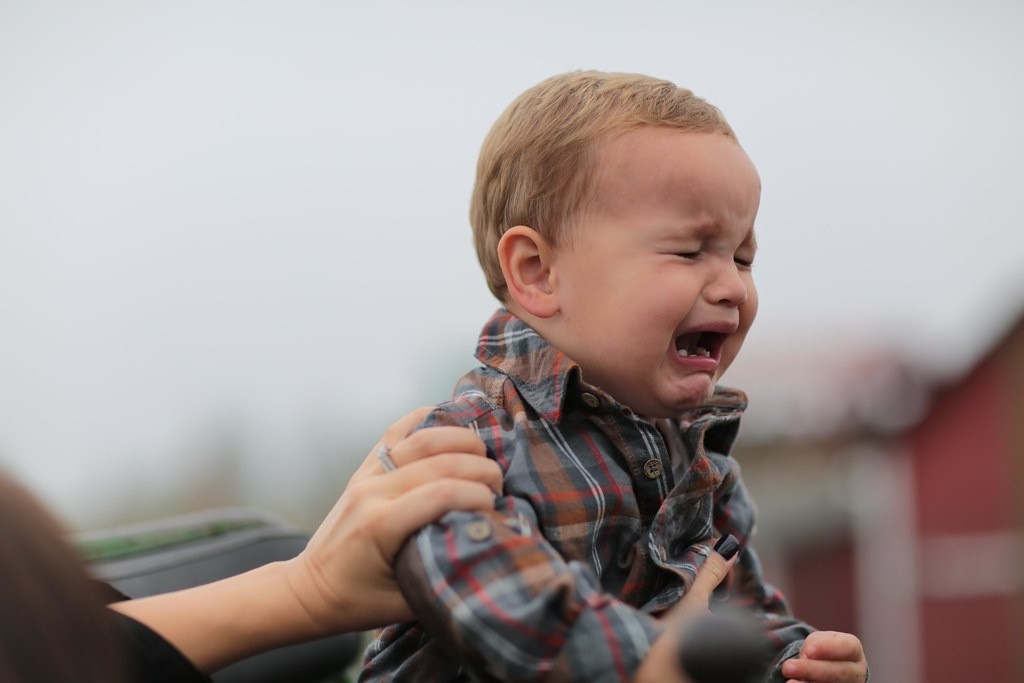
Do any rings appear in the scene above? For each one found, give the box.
[377,445,396,474]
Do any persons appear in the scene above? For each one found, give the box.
[342,67,873,683]
[0,405,742,683]
